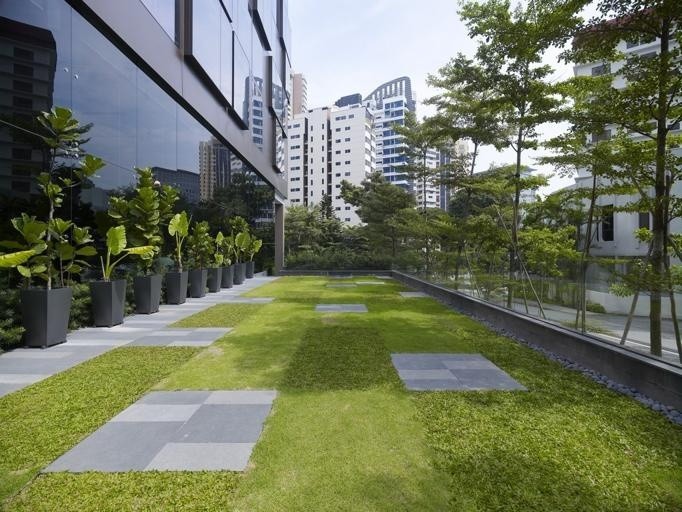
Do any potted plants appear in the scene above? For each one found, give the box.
[165,209,191,304]
[1,105,106,348]
[89,225,159,327]
[188,215,263,297]
[108,164,181,314]
